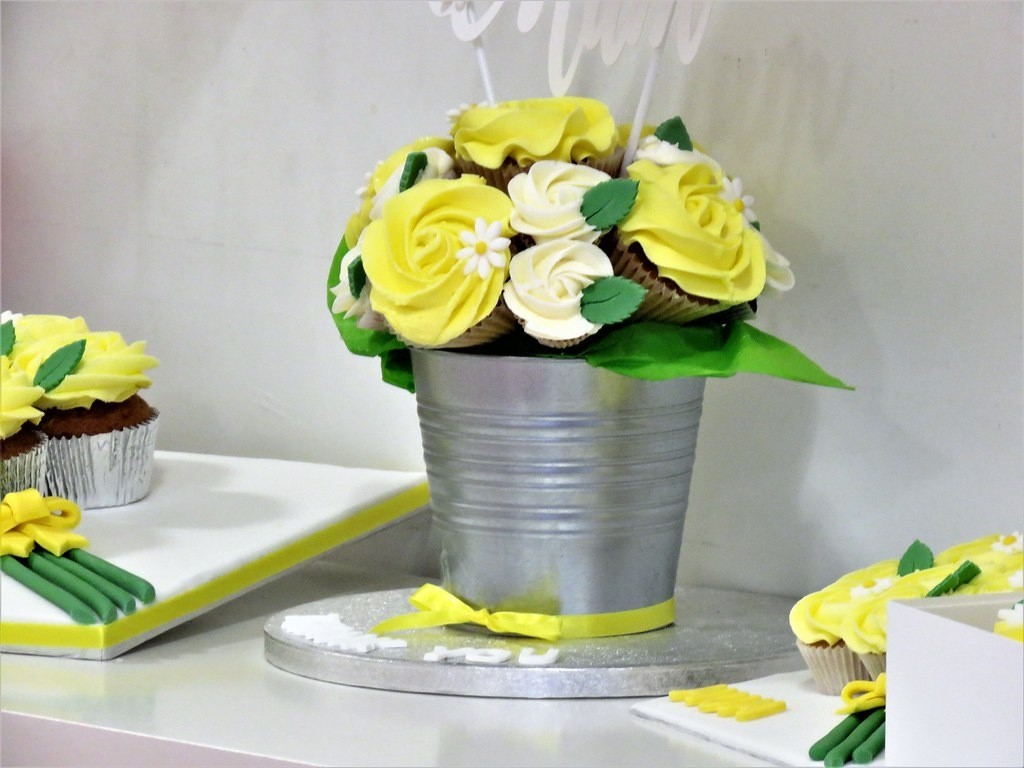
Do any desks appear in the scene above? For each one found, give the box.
[0,567,887,768]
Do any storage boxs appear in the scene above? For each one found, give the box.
[885,590,1023,767]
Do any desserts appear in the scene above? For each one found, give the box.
[789,531,1023,694]
[327,94,794,356]
[0,309,162,508]
